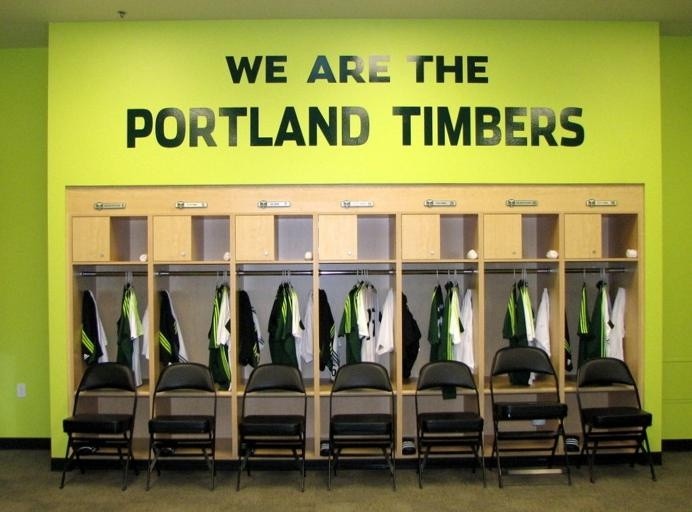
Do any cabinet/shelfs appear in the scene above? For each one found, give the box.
[399,184,481,454]
[67,185,150,461]
[149,185,235,462]
[315,183,399,461]
[562,184,645,447]
[481,184,561,455]
[235,185,315,461]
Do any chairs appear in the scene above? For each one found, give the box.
[490,347,573,488]
[60,363,137,491]
[237,364,307,493]
[576,358,656,482]
[147,362,217,491]
[328,362,396,492]
[415,360,487,487]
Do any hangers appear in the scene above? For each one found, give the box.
[355,267,371,286]
[280,267,292,286]
[511,265,551,290]
[124,270,134,292]
[580,267,627,288]
[436,267,475,288]
[215,270,230,288]
